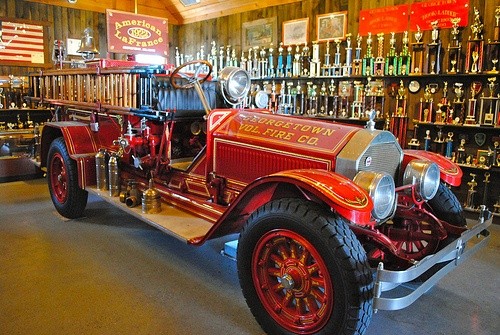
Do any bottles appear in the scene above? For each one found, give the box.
[95,149,121,198]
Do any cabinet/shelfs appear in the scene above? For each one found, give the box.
[0,67,500,224]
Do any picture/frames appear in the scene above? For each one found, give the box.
[241,15,278,52]
[315,10,348,44]
[280,16,310,47]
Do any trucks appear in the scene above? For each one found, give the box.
[27,58,495,335]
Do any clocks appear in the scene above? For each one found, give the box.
[408,78,420,92]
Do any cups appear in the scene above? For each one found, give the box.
[125,196,137,208]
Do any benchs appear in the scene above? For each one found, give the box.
[136,72,242,125]
